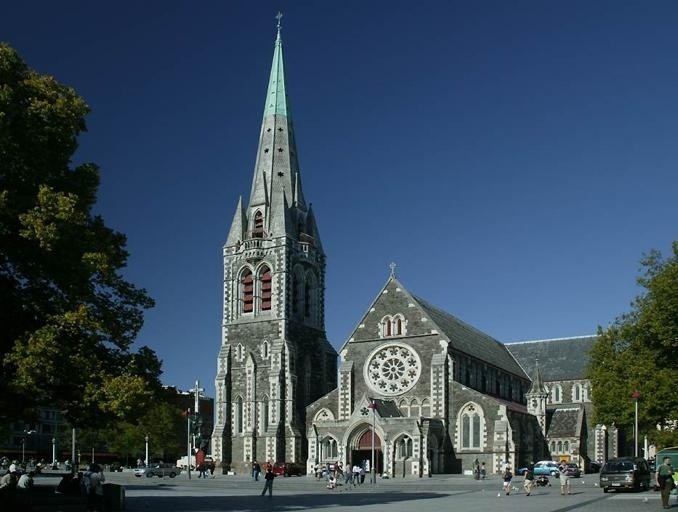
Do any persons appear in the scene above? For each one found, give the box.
[523,466,532,496]
[558,460,571,495]
[501,466,512,496]
[194,459,366,498]
[0,454,107,512]
[472,457,479,481]
[653,457,675,509]
[480,462,486,479]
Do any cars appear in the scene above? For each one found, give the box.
[519,460,582,478]
[133,462,181,479]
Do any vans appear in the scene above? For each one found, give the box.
[272,462,303,477]
[599,457,650,493]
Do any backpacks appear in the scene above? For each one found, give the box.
[81,473,93,494]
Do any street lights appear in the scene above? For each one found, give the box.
[24,428,38,467]
[632,389,641,457]
[51,438,56,467]
[182,407,192,479]
[367,400,380,484]
[144,433,150,467]
[20,437,27,464]
